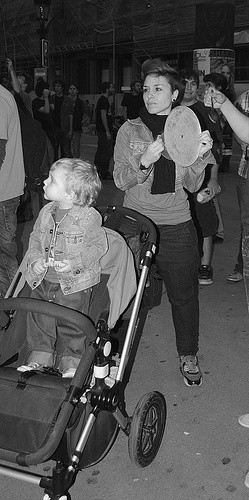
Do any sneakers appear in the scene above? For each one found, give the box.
[178,354,203,388]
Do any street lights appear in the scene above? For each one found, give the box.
[84,0,118,128]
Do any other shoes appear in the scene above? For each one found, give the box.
[226,269,243,282]
[15,361,54,374]
[198,263,213,285]
[238,412,249,427]
[61,367,77,379]
[244,471,249,488]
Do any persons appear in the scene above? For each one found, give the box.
[113,58,216,388]
[179,68,249,310]
[0,58,145,298]
[16,157,109,379]
[214,63,235,93]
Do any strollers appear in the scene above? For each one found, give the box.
[0,206,168,500]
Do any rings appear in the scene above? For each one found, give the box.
[210,140,212,142]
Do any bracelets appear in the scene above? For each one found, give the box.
[140,164,149,171]
[45,96,48,98]
